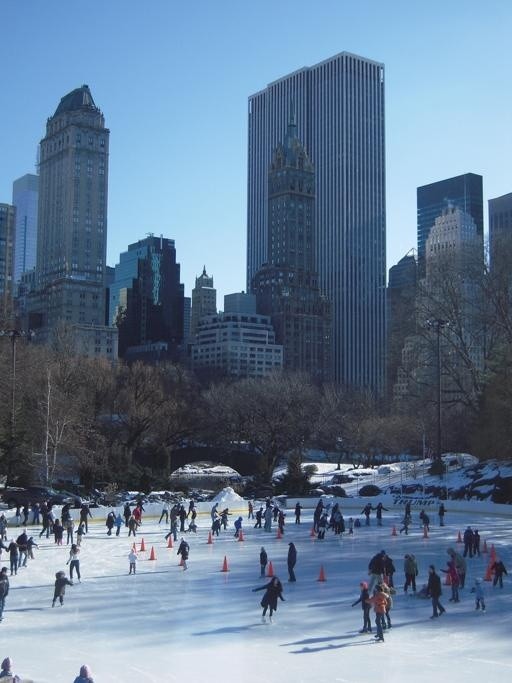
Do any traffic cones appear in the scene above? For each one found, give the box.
[444,572,451,585]
[178,556,184,566]
[206,531,213,544]
[317,565,325,581]
[483,541,497,581]
[391,526,397,536]
[266,560,274,577]
[133,543,137,555]
[167,535,172,548]
[140,538,145,551]
[149,546,157,560]
[423,526,429,537]
[455,531,462,543]
[220,555,230,571]
[237,529,244,541]
[310,528,314,536]
[275,528,282,538]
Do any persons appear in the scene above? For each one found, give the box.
[1,656,95,683]
[208,499,287,538]
[427,526,508,618]
[350,548,418,642]
[104,498,197,575]
[1,500,94,609]
[294,499,391,539]
[251,542,297,625]
[399,501,448,536]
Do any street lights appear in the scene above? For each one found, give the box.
[1,329,34,486]
[422,320,449,460]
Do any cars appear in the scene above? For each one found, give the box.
[2,481,214,508]
